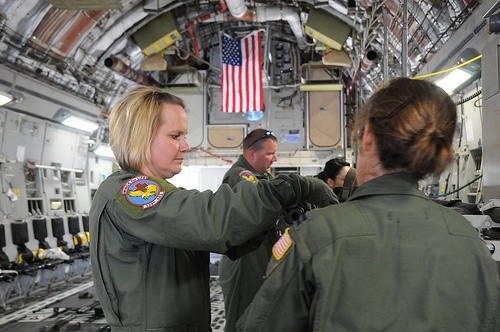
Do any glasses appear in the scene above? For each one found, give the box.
[247,131,274,149]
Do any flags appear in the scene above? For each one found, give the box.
[219,30,264,113]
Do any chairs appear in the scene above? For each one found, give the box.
[0,215,91,310]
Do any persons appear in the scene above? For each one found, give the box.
[89,86,339,332]
[234,77,500,332]
[218,129,351,332]
